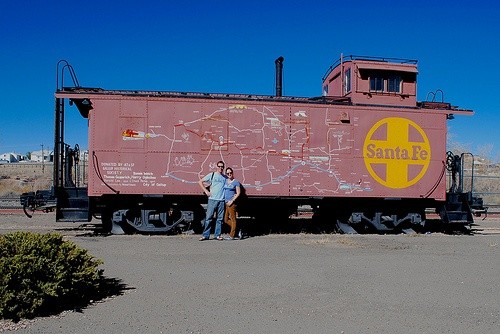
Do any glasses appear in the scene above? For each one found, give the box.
[226,172,232,175]
[218,166,223,168]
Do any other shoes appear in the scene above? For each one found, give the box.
[198,237,205,241]
[239,230,242,240]
[217,237,223,240]
[224,238,234,240]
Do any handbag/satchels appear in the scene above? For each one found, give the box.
[203,172,215,194]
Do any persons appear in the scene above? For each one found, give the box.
[208,167,244,241]
[199,161,227,241]
